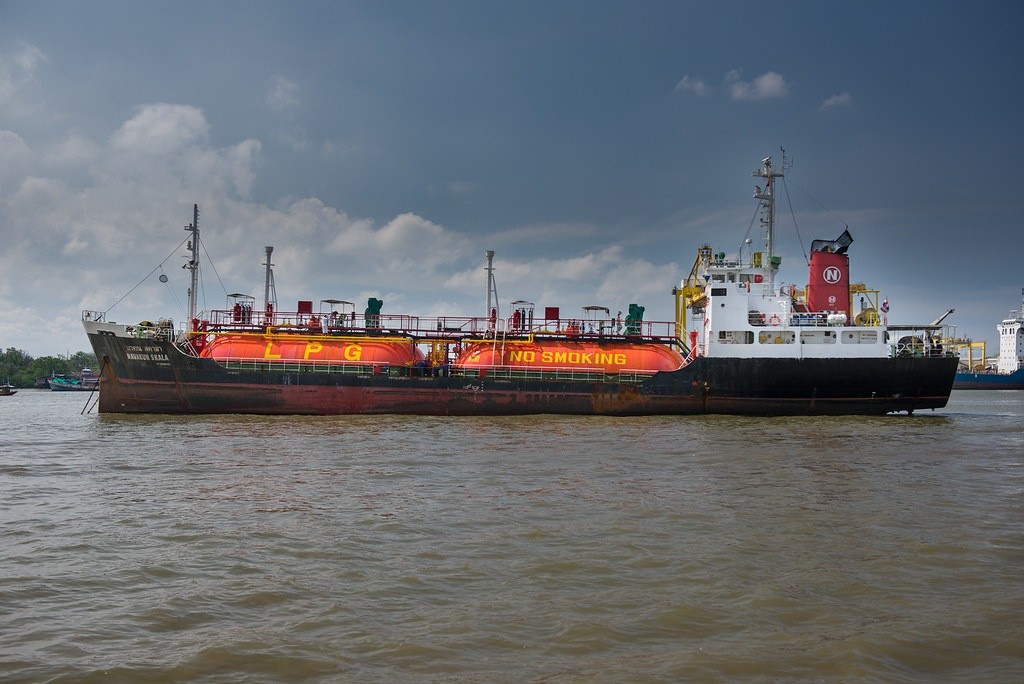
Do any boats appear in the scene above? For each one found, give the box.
[0,383,18,396]
[48,372,100,391]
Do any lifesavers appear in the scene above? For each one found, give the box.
[769,315,780,327]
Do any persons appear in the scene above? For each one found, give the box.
[322,314,329,334]
[927,336,935,355]
[616,310,623,336]
[935,341,944,355]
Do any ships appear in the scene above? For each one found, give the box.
[82,143,960,416]
